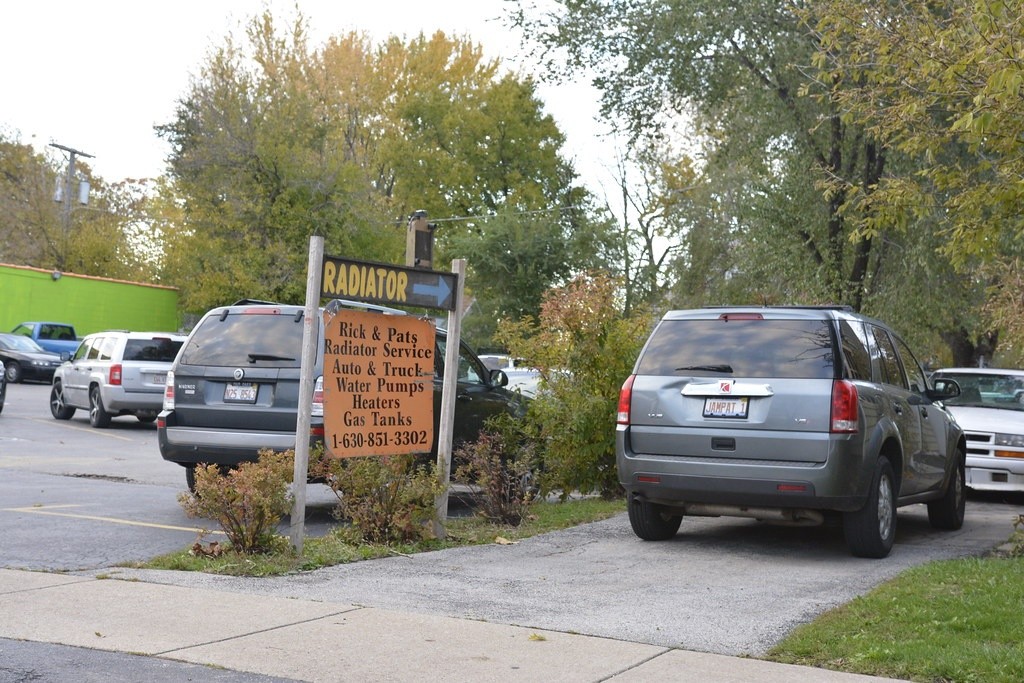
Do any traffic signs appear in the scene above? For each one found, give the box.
[319,252,461,312]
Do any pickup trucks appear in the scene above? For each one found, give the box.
[11,321,83,356]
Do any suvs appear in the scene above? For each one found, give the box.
[156,297,542,504]
[49,329,189,429]
[614,304,967,560]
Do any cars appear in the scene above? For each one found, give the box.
[0,331,65,385]
[467,354,574,399]
[926,367,1024,494]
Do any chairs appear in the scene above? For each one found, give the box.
[950,387,982,404]
